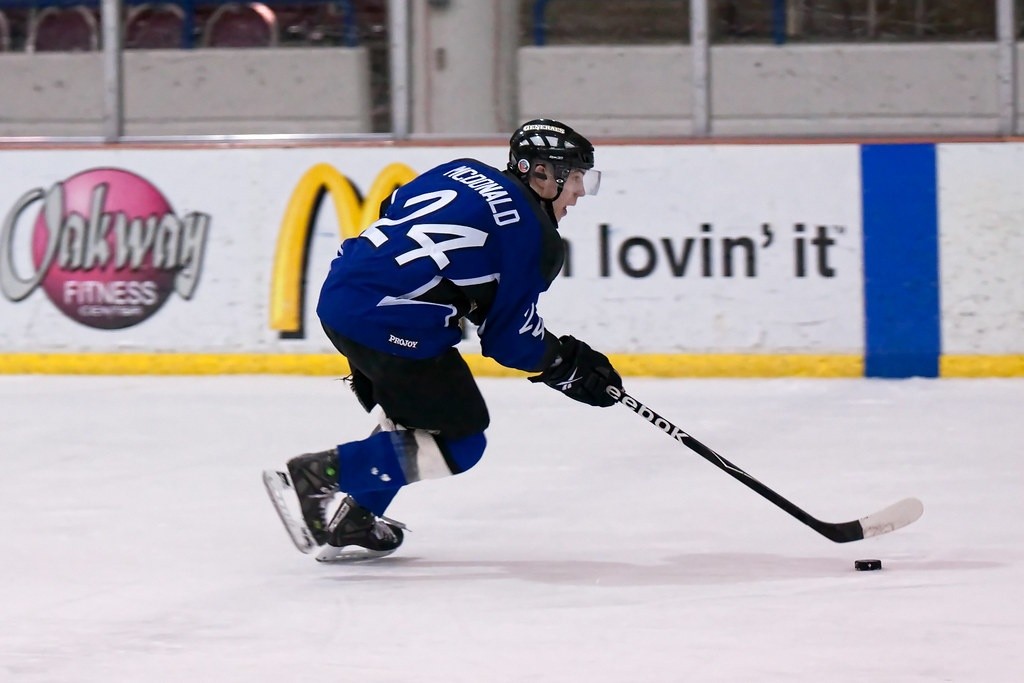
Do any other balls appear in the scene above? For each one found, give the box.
[854,558,882,571]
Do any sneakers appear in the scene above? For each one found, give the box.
[263,449,343,555]
[315,496,413,562]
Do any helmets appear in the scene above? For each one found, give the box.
[507,119,594,170]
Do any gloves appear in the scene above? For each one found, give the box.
[527,335,625,407]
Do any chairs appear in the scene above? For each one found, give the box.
[121,3,185,49]
[200,0,279,48]
[0,12,11,51]
[24,5,99,52]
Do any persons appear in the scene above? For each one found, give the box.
[285,119,622,551]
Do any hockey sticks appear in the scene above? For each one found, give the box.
[619,390,927,544]
